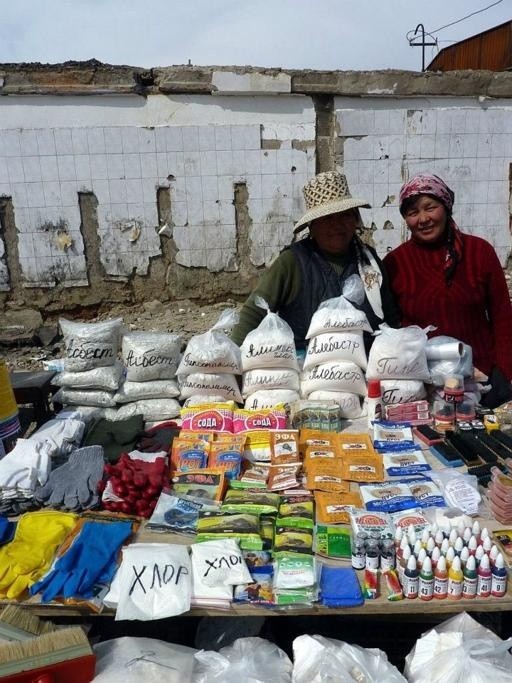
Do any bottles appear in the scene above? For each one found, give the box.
[352,519,509,602]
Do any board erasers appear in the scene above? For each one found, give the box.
[412,424,512,487]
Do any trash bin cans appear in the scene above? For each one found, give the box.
[12,371,66,431]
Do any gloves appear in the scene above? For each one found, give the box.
[0,416,177,606]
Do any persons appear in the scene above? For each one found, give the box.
[381,171,512,410]
[226,171,398,357]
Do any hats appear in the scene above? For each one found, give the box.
[292,170,372,232]
